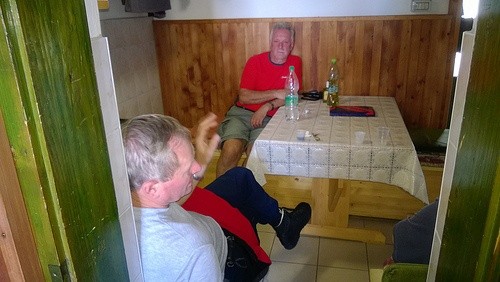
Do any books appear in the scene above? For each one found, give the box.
[330,106,375,117]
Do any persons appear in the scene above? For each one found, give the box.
[216,22,302,179]
[121,114,311,282]
[383,197,439,265]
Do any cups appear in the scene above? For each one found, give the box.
[295,129,306,141]
[377,127,391,146]
[354,131,366,144]
[302,104,312,120]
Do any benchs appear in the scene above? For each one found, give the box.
[190,126,453,220]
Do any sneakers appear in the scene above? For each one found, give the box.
[273,202,312,249]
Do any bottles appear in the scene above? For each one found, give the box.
[285,66,299,122]
[327,59,340,105]
[323,80,329,103]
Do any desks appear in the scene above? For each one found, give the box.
[246,96,429,243]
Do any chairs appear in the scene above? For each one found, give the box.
[381,262,428,282]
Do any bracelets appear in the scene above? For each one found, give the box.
[192,174,204,181]
[269,101,274,110]
